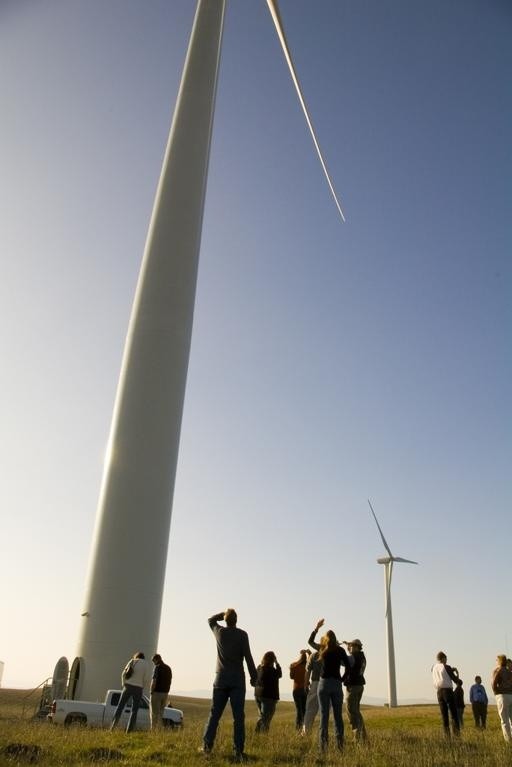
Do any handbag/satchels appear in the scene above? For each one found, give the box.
[125,663,134,679]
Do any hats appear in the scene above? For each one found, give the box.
[348,639,362,646]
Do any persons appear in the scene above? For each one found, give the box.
[250,651,281,733]
[290,618,369,752]
[433,652,511,743]
[150,654,172,731]
[111,652,148,734]
[198,609,258,757]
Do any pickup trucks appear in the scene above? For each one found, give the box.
[44,690,186,736]
